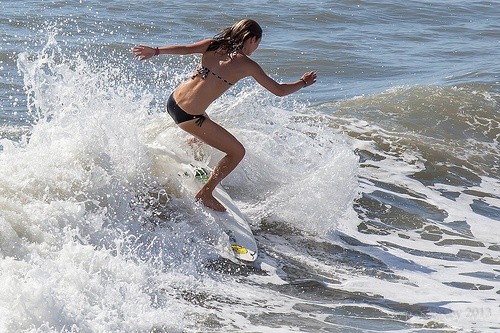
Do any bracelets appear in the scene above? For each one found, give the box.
[299,77,308,88]
[152,46,162,57]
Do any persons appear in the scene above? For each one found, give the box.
[133,19,317,211]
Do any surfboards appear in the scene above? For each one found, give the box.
[175,160,258,263]
